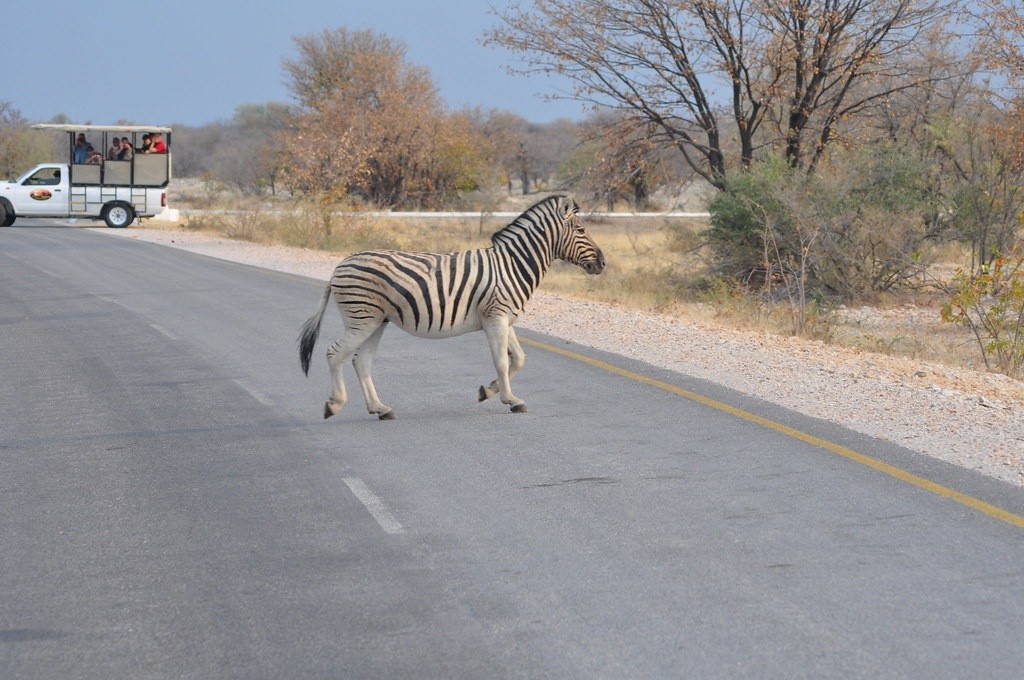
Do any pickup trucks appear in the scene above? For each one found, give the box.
[0,124,172,228]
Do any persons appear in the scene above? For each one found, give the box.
[37,171,61,184]
[72,134,102,165]
[108,137,132,160]
[142,133,166,154]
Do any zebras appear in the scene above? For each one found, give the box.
[295,193,607,422]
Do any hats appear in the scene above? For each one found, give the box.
[86,147,94,152]
